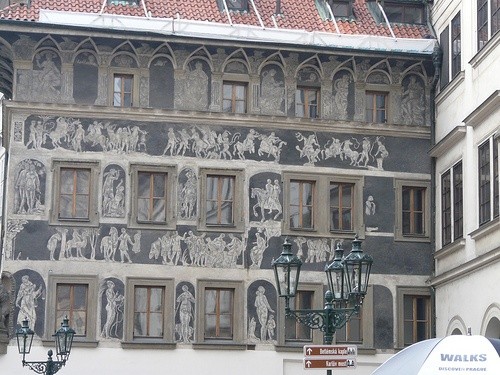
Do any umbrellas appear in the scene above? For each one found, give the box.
[370,327,500,375]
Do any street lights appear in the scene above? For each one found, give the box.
[15,314,76,375]
[271,234,374,375]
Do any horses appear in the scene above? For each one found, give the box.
[258,135,278,160]
[340,140,359,167]
[41,117,71,148]
[194,129,220,159]
[66,231,89,259]
[321,138,342,161]
[250,186,284,221]
[84,124,105,150]
[233,132,255,161]
[99,224,120,264]
[128,125,140,152]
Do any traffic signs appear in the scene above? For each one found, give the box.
[303,344,358,358]
[303,358,356,370]
